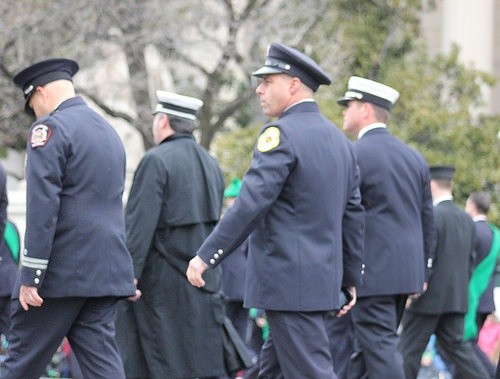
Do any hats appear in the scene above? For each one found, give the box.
[251,41,332,92]
[337,76,400,111]
[13,58,79,116]
[152,90,204,121]
[429,168,455,179]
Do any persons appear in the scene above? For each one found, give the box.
[0,56,136,379]
[186,43,364,379]
[337,75,434,379]
[114,90,225,379]
[0,162,500,379]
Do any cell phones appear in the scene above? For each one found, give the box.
[327,286,353,318]
